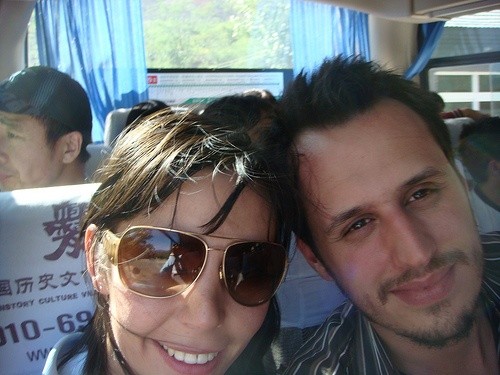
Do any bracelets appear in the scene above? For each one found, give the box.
[452,108,465,119]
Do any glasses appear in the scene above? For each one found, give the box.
[98,225,290,309]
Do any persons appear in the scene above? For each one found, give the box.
[42,107,302,375]
[0,66,92,193]
[42,55,500,375]
[264,52,500,375]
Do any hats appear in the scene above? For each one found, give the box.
[2,65,92,134]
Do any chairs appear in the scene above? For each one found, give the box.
[0,109,500,375]
